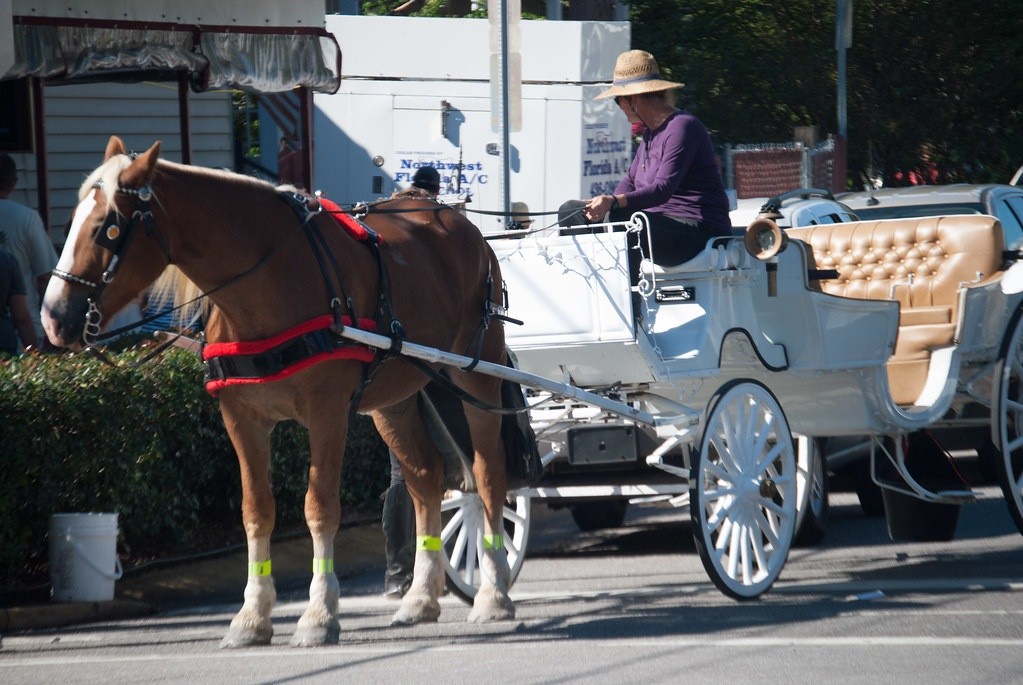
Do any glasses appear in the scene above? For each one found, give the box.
[614,96,623,105]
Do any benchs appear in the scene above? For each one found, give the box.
[777,215,1006,403]
[636,235,754,363]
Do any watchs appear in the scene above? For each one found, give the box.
[611,195,619,211]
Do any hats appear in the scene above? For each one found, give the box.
[593,49,684,100]
[498,201,534,226]
[415,166,440,186]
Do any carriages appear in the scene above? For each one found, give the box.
[39,134,1023,649]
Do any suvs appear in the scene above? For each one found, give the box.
[830,183,1023,256]
[722,186,863,234]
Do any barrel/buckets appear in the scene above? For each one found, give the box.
[48,511,124,602]
[879,478,968,544]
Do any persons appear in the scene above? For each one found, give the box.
[582,49,734,342]
[0,152,61,359]
[98,279,210,346]
[410,166,441,203]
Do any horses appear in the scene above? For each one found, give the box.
[37,138,514,648]
